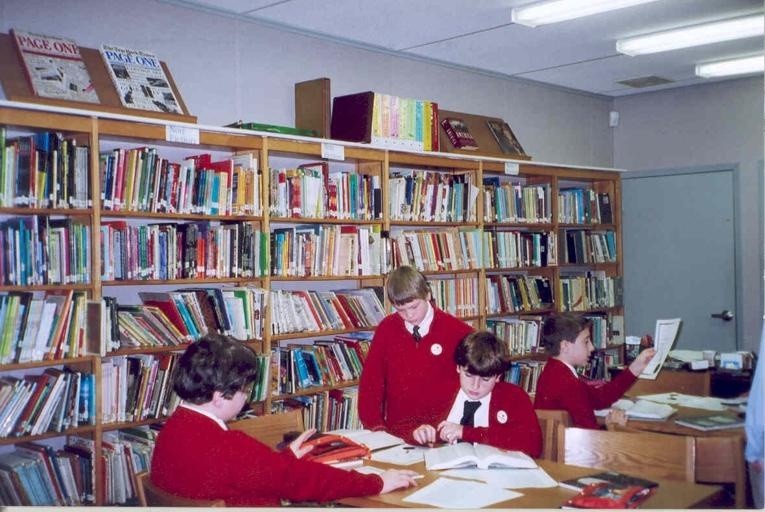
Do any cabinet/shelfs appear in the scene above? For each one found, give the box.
[559,164,627,384]
[100,112,266,507]
[0,100,100,506]
[482,155,558,407]
[386,151,482,338]
[267,134,386,448]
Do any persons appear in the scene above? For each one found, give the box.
[744,321,765,509]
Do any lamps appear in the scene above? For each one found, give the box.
[509,1,650,35]
[613,10,765,58]
[694,49,765,84]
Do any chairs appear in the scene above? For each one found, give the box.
[228,410,304,451]
[608,422,745,507]
[624,372,710,400]
[535,410,568,464]
[135,471,226,507]
[563,426,696,485]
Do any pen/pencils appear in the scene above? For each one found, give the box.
[412,475,424,479]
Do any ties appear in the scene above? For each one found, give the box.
[459,399,482,426]
[412,325,421,343]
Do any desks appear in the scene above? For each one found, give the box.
[337,442,723,509]
[621,403,745,438]
